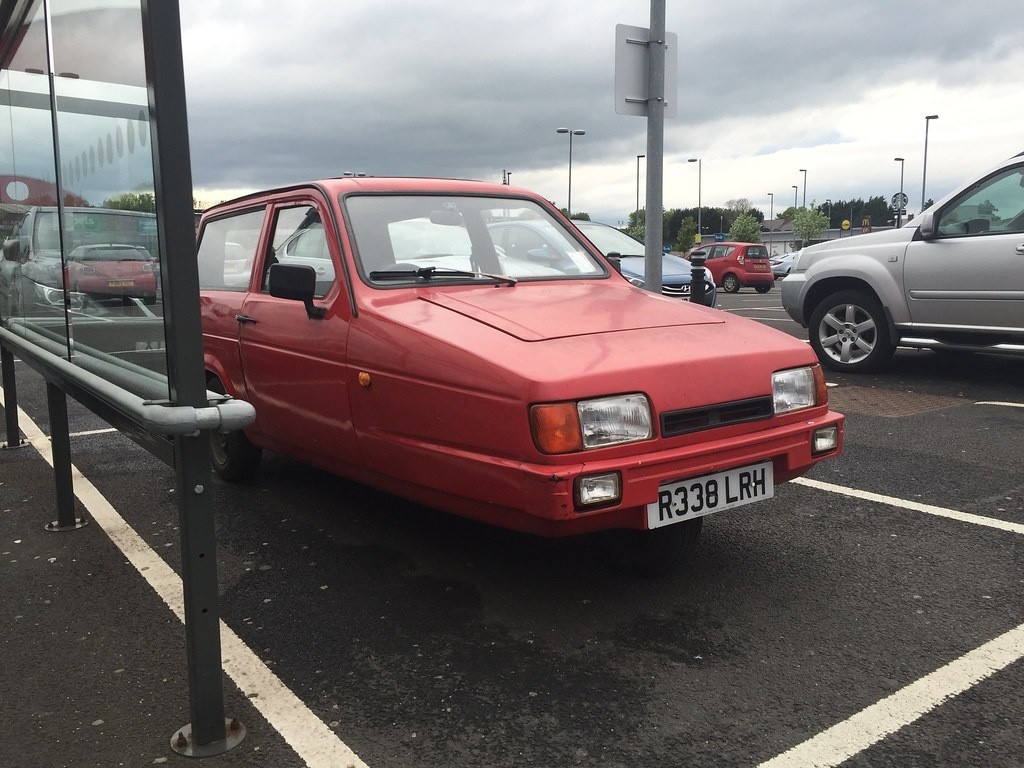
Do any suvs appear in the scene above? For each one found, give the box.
[779,146,1024,374]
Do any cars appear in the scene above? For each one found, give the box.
[485,220,716,308]
[55,242,158,306]
[136,245,160,290]
[0,206,154,324]
[224,242,251,289]
[275,217,567,278]
[201,175,845,561]
[768,251,800,280]
[683,241,775,294]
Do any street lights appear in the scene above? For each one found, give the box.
[507,172,512,185]
[687,158,702,233]
[767,193,775,257]
[636,154,645,213]
[792,185,798,210]
[825,199,832,241]
[556,128,586,216]
[799,169,808,209]
[26,66,78,360]
[893,157,905,226]
[921,114,940,211]
